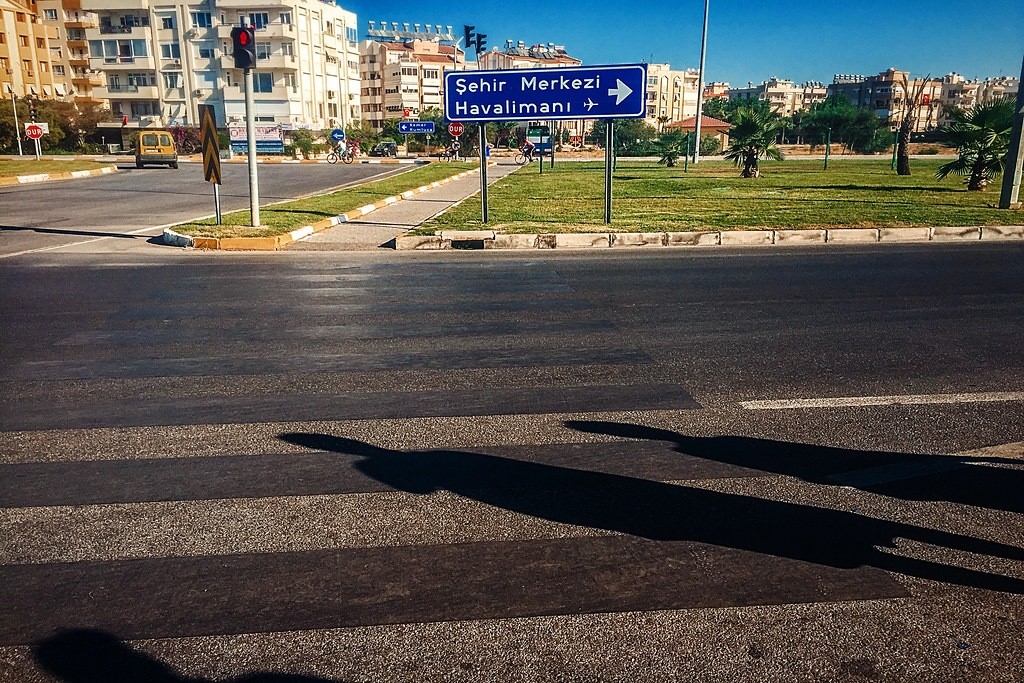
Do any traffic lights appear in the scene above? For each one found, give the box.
[228,26,259,71]
[29,103,37,121]
[463,24,476,48]
[475,33,488,54]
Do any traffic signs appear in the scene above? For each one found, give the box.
[444,63,648,123]
[399,121,435,134]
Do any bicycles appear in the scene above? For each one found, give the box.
[438,149,466,164]
[327,148,353,165]
[515,146,539,165]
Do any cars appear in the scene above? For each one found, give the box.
[372,142,398,157]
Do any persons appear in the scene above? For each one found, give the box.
[444,139,456,159]
[485,142,494,170]
[332,136,361,161]
[519,135,536,162]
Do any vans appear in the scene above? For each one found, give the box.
[135,130,178,169]
